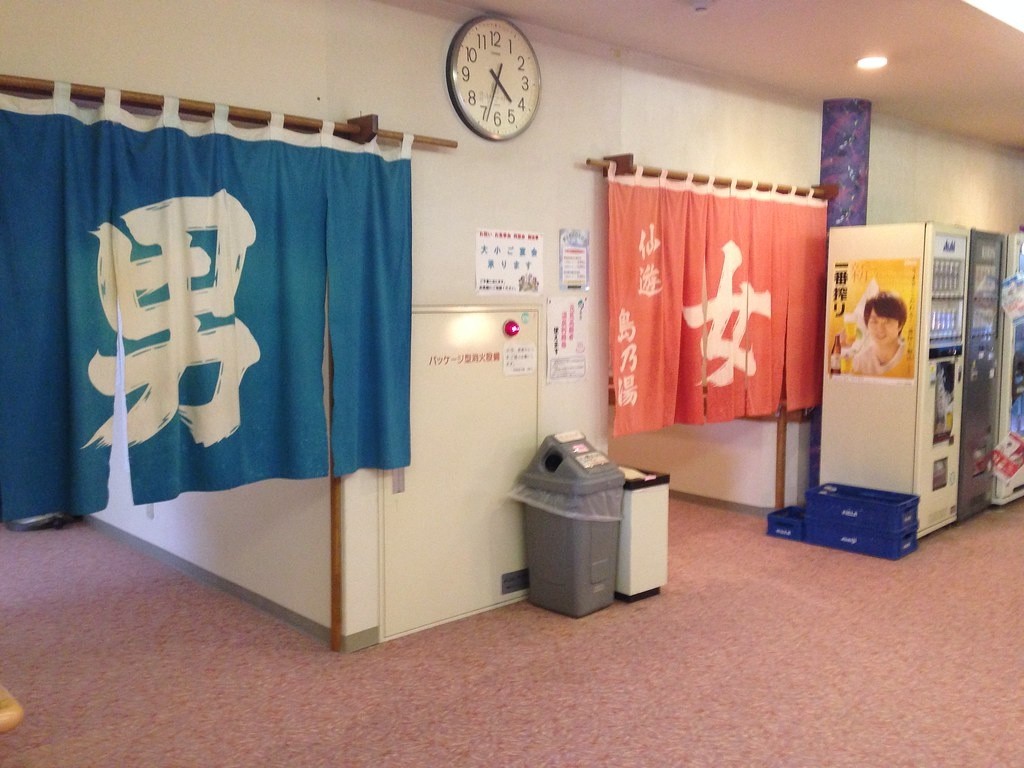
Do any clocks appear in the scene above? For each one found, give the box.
[447,15,542,142]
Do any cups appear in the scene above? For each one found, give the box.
[843,313,858,344]
[841,348,854,374]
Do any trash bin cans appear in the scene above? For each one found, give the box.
[615,464,670,605]
[516,430,626,619]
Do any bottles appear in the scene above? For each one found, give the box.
[830,335,841,373]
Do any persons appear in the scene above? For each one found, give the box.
[849,291,914,379]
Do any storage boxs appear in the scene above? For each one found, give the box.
[766,483,920,561]
